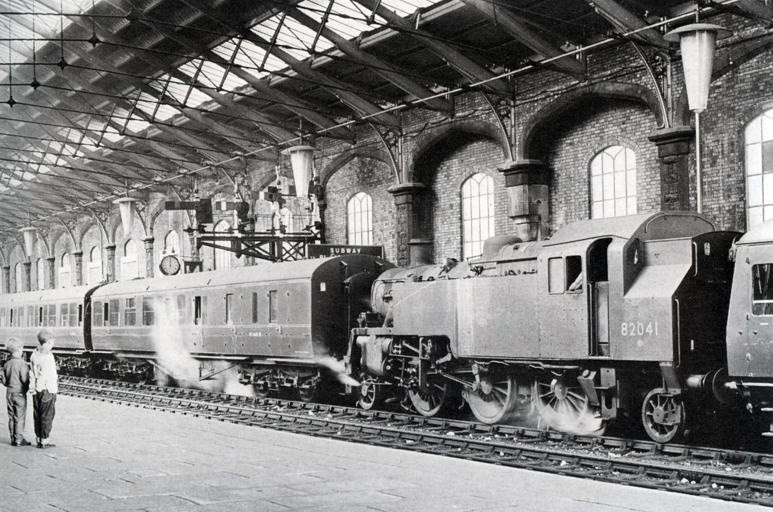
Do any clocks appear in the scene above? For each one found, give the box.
[159,255,181,275]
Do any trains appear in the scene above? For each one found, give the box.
[0,211,772,443]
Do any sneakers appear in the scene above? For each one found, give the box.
[10,439,55,448]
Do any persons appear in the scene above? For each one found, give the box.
[29,328,60,449]
[0,336,32,446]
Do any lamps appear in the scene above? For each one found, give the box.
[280,116,321,196]
[663,7,733,113]
[18,210,40,257]
[112,179,140,230]
